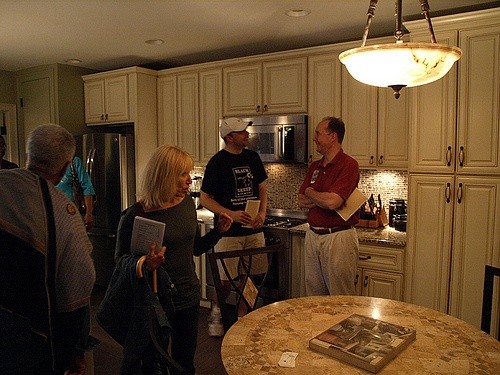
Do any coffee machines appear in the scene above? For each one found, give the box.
[389,199,408,232]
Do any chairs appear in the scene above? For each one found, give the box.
[208,245,285,332]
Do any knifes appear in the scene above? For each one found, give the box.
[368,193,382,215]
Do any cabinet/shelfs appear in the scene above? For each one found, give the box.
[158,33,408,171]
[82,67,134,124]
[402,8,500,340]
[293,237,403,301]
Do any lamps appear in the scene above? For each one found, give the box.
[338,0,463,99]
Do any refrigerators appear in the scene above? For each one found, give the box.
[73,131,135,308]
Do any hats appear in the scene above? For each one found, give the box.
[220,118,253,138]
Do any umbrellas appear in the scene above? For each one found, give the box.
[136,256,171,375]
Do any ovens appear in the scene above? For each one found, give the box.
[204,225,292,317]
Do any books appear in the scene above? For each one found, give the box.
[309,314,416,374]
[334,187,367,220]
[241,200,261,229]
[130,216,165,256]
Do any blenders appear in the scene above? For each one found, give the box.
[189,176,203,209]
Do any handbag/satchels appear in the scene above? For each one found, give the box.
[71,159,95,215]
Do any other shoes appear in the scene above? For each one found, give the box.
[209,307,224,336]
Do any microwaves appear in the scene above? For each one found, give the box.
[217,113,309,164]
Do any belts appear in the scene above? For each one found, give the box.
[310,226,353,235]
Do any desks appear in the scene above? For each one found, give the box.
[221,295,500,375]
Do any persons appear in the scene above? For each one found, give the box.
[0,135,18,169]
[114,144,234,375]
[56,156,96,231]
[200,117,268,337]
[297,117,360,296]
[0,124,97,375]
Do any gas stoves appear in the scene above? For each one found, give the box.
[258,208,308,249]
